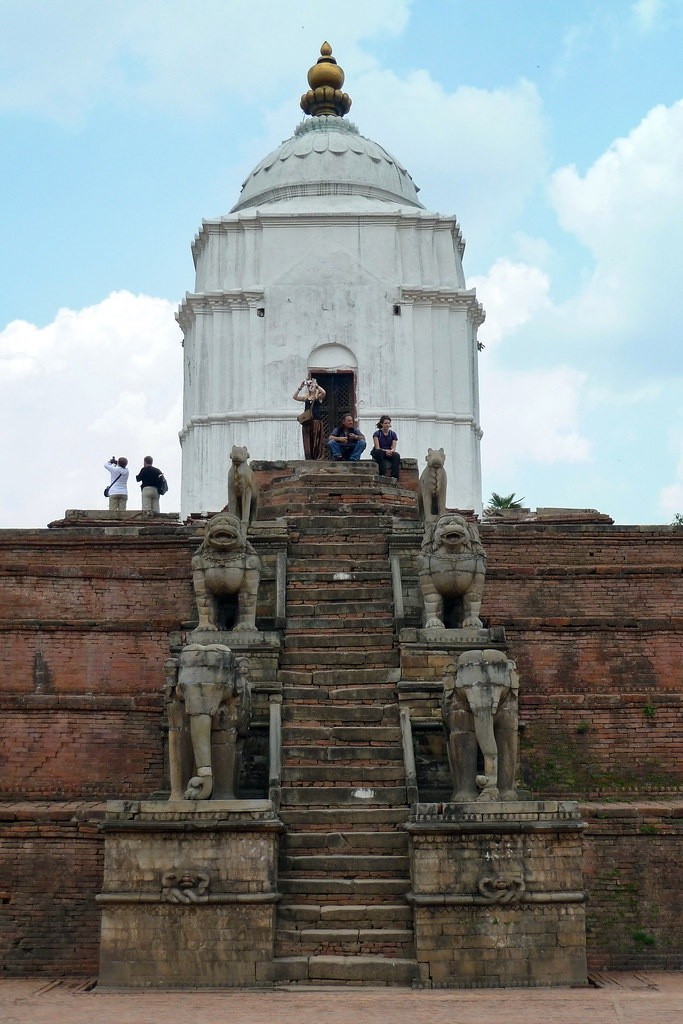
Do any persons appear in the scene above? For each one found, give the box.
[136,456,162,513]
[370,416,400,479]
[104,457,129,510]
[328,413,367,461]
[293,381,326,460]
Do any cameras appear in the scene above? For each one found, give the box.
[110,456,118,465]
[304,380,311,385]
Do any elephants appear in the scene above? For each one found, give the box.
[165,644,254,800]
[442,649,522,803]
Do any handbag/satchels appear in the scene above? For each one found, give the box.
[104,486,110,497]
[297,409,313,425]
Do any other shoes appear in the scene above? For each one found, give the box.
[333,456,345,461]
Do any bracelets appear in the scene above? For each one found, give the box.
[298,388,301,390]
[316,386,318,389]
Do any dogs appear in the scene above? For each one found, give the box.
[227,445,260,522]
[418,447,447,522]
